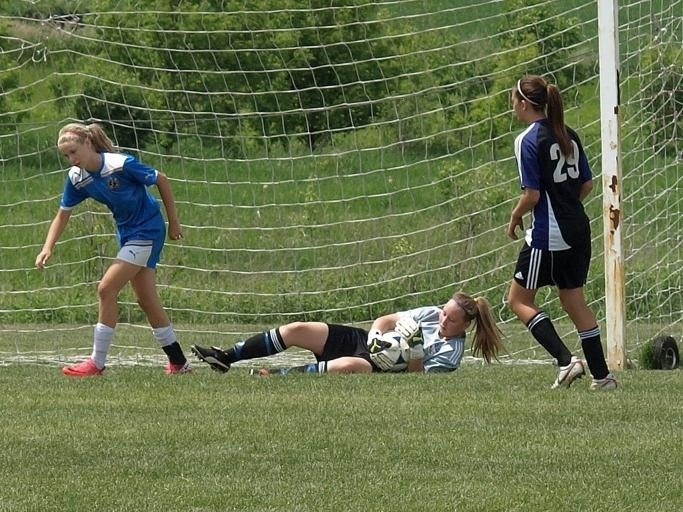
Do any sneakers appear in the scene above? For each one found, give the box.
[590,373,617,391]
[551,356,585,390]
[166,362,191,374]
[62,359,105,376]
[191,344,231,373]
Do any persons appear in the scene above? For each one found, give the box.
[506,74,617,393]
[190,293,515,375]
[35,121,191,377]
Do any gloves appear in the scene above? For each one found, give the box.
[394,317,424,359]
[367,332,398,371]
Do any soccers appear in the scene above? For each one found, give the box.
[378,331,409,371]
